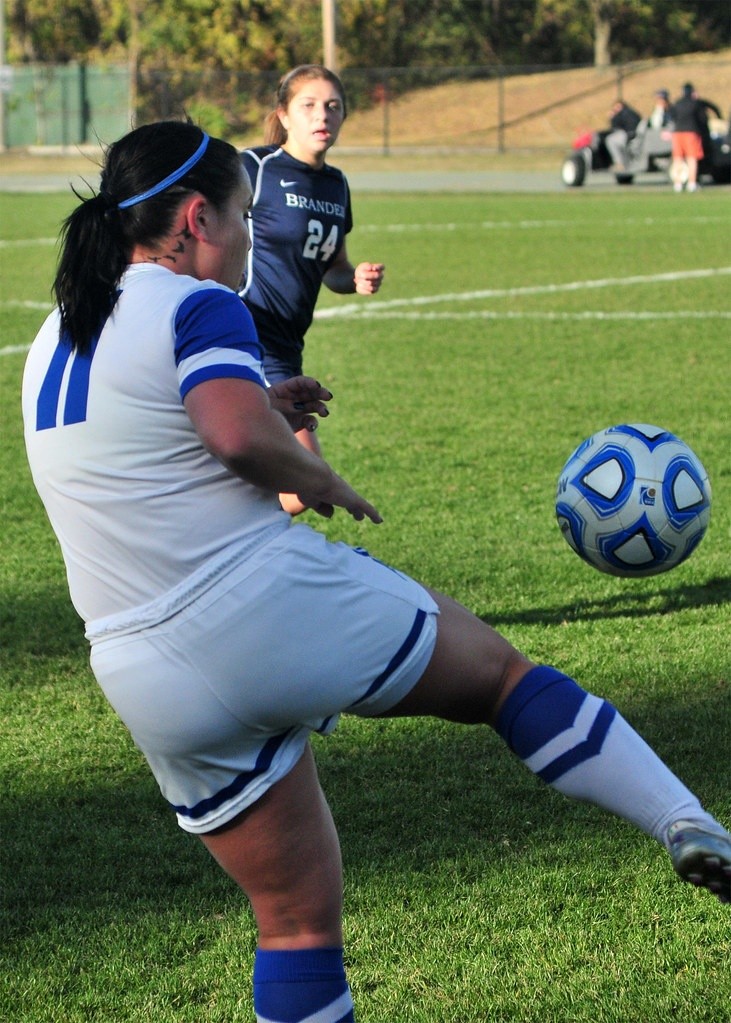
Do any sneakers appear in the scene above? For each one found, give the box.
[669,828,731,905]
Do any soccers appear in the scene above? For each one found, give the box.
[557,426,712,578]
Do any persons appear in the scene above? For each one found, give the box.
[22,121,728,1023]
[234,63,387,521]
[603,100,643,172]
[641,80,726,195]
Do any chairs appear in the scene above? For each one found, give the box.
[580,122,731,186]
